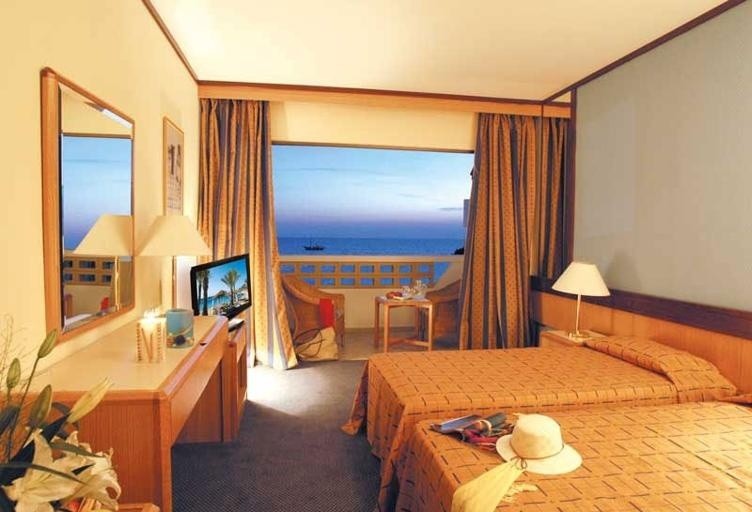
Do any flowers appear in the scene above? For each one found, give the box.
[0,310,123,512]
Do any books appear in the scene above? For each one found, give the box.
[430,410,515,454]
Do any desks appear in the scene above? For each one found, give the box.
[1,313,232,512]
[178,319,249,445]
[119,502,160,512]
[374,295,433,352]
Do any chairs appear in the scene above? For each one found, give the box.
[420,269,463,340]
[281,275,345,358]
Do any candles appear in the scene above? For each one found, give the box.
[133,316,168,362]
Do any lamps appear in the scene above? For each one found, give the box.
[136,215,212,312]
[71,214,134,313]
[551,262,611,340]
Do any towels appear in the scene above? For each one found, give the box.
[319,297,335,329]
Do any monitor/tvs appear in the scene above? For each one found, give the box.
[190,253,251,331]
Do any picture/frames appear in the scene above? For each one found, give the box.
[162,115,185,215]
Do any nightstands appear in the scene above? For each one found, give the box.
[539,328,605,346]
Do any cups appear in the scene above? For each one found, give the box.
[166,308,193,348]
[402,286,409,296]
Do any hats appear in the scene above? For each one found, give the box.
[496,414,583,476]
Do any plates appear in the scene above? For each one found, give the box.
[393,295,411,299]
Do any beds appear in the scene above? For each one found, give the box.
[367,332,737,422]
[411,398,752,512]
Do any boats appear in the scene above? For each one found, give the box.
[302,239,327,252]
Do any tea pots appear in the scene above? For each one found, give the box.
[413,280,426,299]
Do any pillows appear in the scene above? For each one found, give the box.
[583,334,739,405]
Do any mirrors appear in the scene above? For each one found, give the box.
[39,65,135,344]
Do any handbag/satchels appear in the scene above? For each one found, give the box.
[286,327,339,370]
